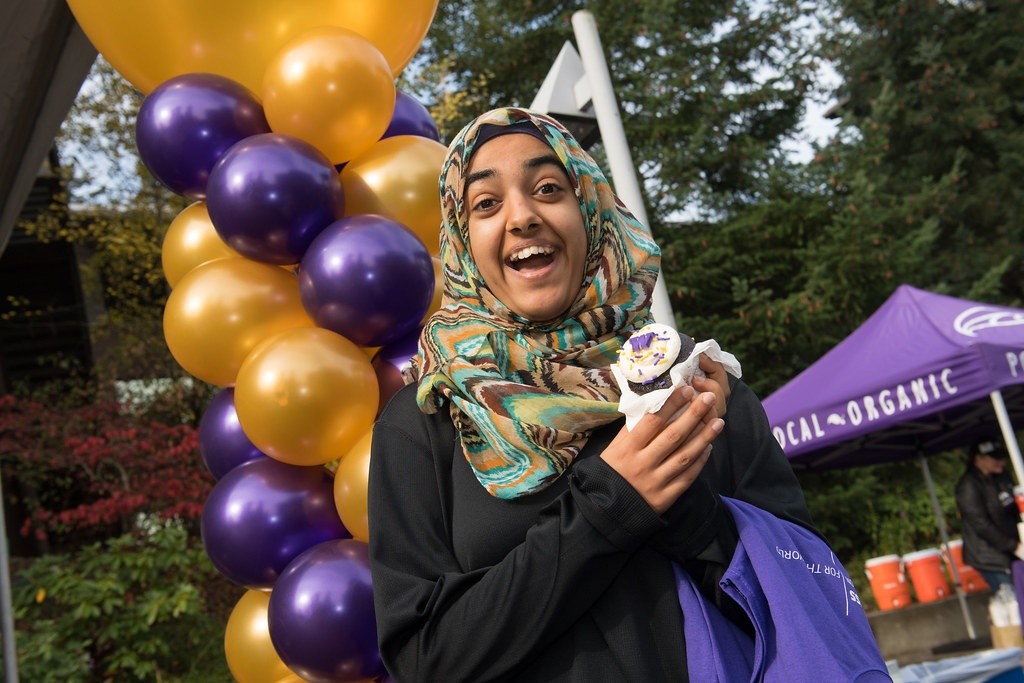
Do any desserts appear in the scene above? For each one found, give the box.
[617,322,695,397]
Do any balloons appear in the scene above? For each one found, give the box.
[67,0,439,100]
[69,26,449,683]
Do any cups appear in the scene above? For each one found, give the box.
[864,554,911,611]
[939,539,989,593]
[902,548,951,602]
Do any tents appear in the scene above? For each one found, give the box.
[759,279,1024,640]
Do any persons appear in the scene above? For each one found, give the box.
[364,106,806,683]
[955,437,1024,593]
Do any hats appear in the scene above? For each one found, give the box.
[970,437,1009,458]
